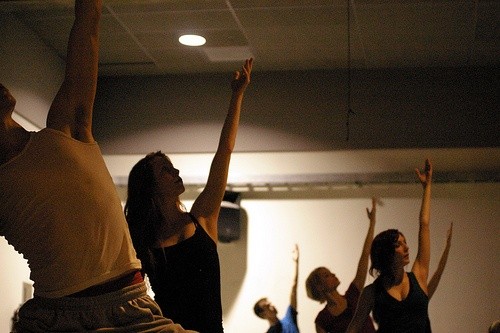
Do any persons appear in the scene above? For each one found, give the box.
[305,194,378,333]
[344,157,433,333]
[0,0,200,333]
[124,56,255,333]
[252,248,301,333]
[426,222,453,302]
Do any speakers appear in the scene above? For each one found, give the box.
[218,190,241,242]
